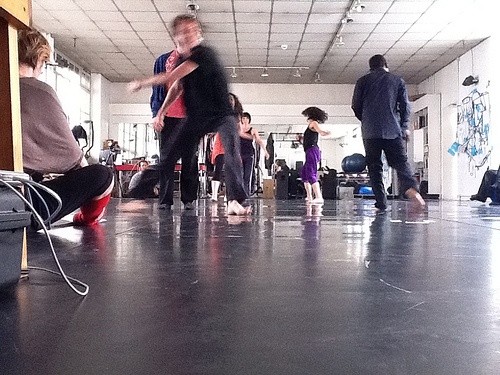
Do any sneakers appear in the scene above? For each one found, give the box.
[228,199,246,215]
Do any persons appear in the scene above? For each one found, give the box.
[210,92,263,201]
[17,27,114,230]
[126,15,248,216]
[294,106,330,205]
[239,112,270,196]
[128,160,160,200]
[351,54,426,210]
[470,163,500,205]
[148,49,206,210]
[272,159,303,200]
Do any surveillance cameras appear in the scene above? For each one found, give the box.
[335,42,344,48]
[260,73,268,77]
[230,72,237,78]
[293,73,302,78]
[315,78,321,83]
[342,17,354,24]
[281,45,287,50]
[357,4,366,13]
[186,3,200,10]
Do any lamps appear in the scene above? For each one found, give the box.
[315,74,323,83]
[230,68,237,78]
[293,69,302,78]
[260,68,269,77]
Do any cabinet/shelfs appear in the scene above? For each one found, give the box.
[404,92,441,200]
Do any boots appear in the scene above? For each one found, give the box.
[210,181,220,202]
[74,195,112,226]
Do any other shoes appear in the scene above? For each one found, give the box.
[472,195,485,202]
[184,202,193,210]
[159,203,171,210]
